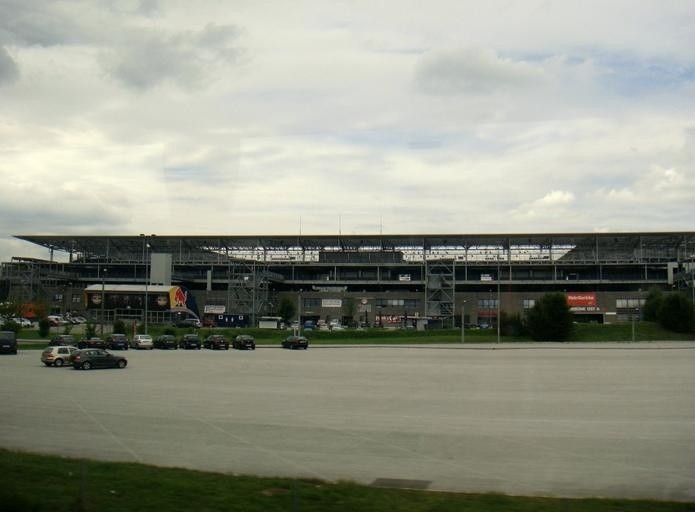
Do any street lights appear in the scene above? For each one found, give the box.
[139,233,155,335]
[100,268,110,336]
[689,253,695,305]
[461,299,469,343]
[637,287,645,320]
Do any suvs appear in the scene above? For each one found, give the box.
[200,318,217,328]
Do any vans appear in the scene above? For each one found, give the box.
[0,331,18,356]
[182,318,203,327]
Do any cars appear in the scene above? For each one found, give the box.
[41,333,130,371]
[382,323,400,331]
[48,312,87,327]
[0,315,33,328]
[399,325,416,330]
[133,334,258,351]
[290,319,384,331]
[281,335,309,349]
